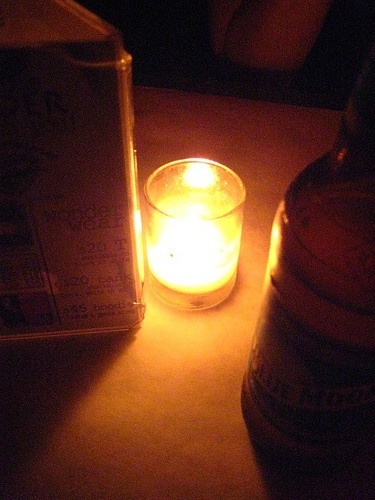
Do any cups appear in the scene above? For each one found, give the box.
[141,158,245,310]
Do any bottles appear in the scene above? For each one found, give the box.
[243,92,375,465]
[3,0,146,341]
